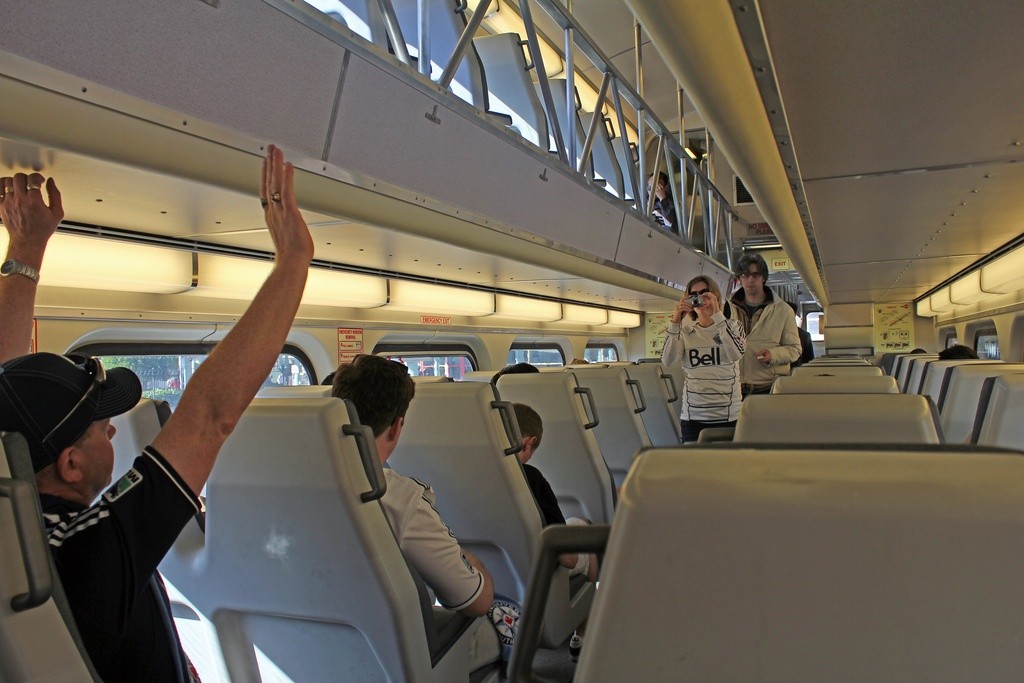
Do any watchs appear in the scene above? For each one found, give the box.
[0,259,40,285]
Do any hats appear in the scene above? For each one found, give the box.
[0,352,142,471]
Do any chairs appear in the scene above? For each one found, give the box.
[322,0,702,250]
[1,333,1024,683]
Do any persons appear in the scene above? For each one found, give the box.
[938,345,980,361]
[910,348,927,354]
[646,170,679,235]
[724,253,802,403]
[783,301,814,370]
[570,358,590,365]
[0,145,319,683]
[499,362,539,378]
[502,397,603,662]
[660,274,746,443]
[322,353,495,617]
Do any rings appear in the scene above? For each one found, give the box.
[4,186,14,193]
[270,191,281,203]
[27,186,40,190]
[0,194,4,198]
[260,197,268,209]
[679,306,682,309]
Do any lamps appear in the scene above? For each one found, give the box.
[456,1,499,19]
[916,243,1024,320]
[0,223,642,330]
[548,59,608,122]
[477,1,563,78]
[604,101,638,149]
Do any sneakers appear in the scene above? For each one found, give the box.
[568,635,584,662]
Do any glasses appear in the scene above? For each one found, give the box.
[739,272,761,279]
[646,184,651,191]
[689,288,709,297]
[42,353,106,442]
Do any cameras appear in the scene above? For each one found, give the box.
[685,295,704,308]
